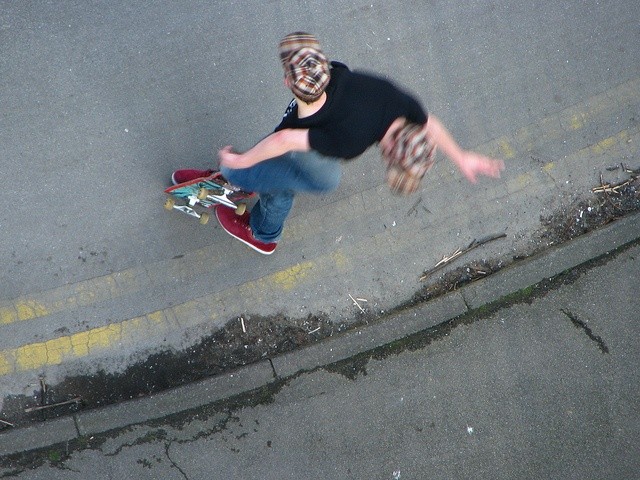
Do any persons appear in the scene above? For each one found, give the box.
[171,31,505,255]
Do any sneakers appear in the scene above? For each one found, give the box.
[216,204,276,255]
[171,170,213,186]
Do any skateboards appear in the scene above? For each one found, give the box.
[163,171,258,226]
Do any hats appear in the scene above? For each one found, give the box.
[386,122,437,195]
[279,31,330,102]
